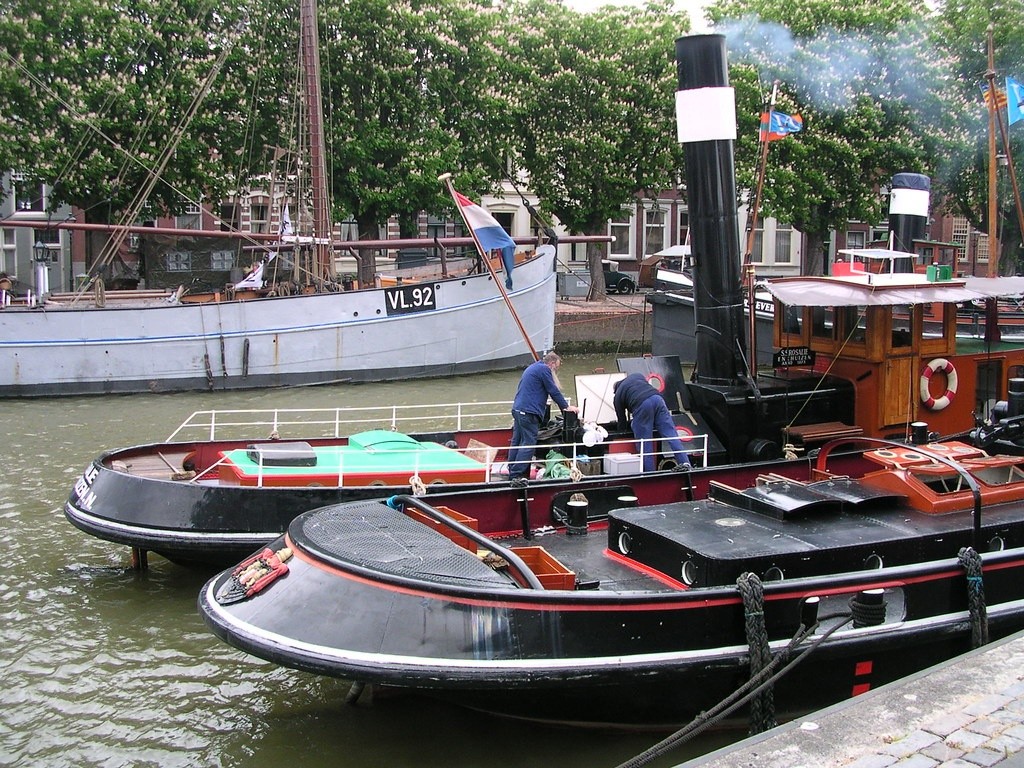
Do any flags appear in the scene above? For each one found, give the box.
[769,111,804,134]
[760,113,802,141]
[1006,75,1024,126]
[455,192,516,294]
[980,81,1008,114]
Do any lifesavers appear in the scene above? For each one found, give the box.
[918,358,957,409]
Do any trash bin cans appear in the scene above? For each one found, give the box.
[557,269,592,302]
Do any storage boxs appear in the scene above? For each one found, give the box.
[508,546,575,591]
[406,505,478,554]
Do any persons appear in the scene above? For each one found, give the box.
[507,351,580,481]
[613,373,693,473]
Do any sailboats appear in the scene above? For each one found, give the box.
[0,0,619,398]
[641,24,1024,368]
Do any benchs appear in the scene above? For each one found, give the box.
[781,421,864,453]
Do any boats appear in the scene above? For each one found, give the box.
[197,377,1024,726]
[65,35,1024,577]
